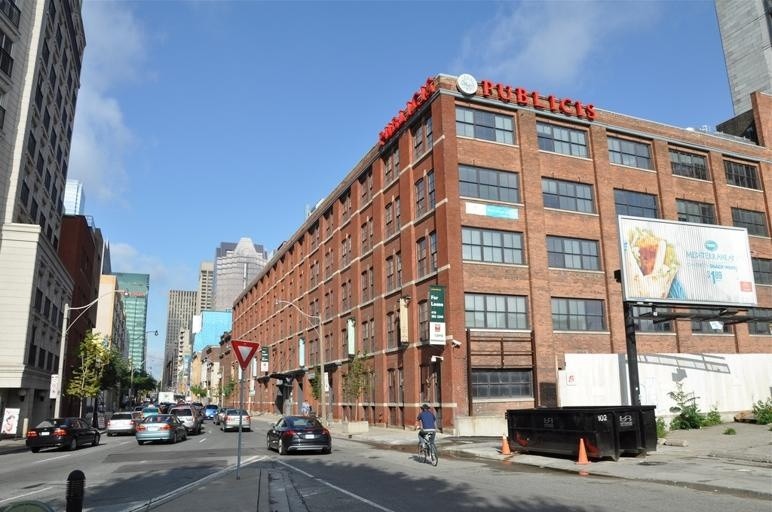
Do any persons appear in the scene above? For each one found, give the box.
[415,404,436,462]
[300,399,311,416]
[5,415,17,432]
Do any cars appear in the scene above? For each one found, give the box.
[106,399,252,445]
[25,416,100,454]
[265,415,332,456]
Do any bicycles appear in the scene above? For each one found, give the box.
[417,432,438,467]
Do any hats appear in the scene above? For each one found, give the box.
[419,403,431,410]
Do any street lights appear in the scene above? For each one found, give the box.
[131,329,158,395]
[274,298,327,428]
[53,289,130,424]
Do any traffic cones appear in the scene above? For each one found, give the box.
[500,434,514,455]
[574,439,594,464]
